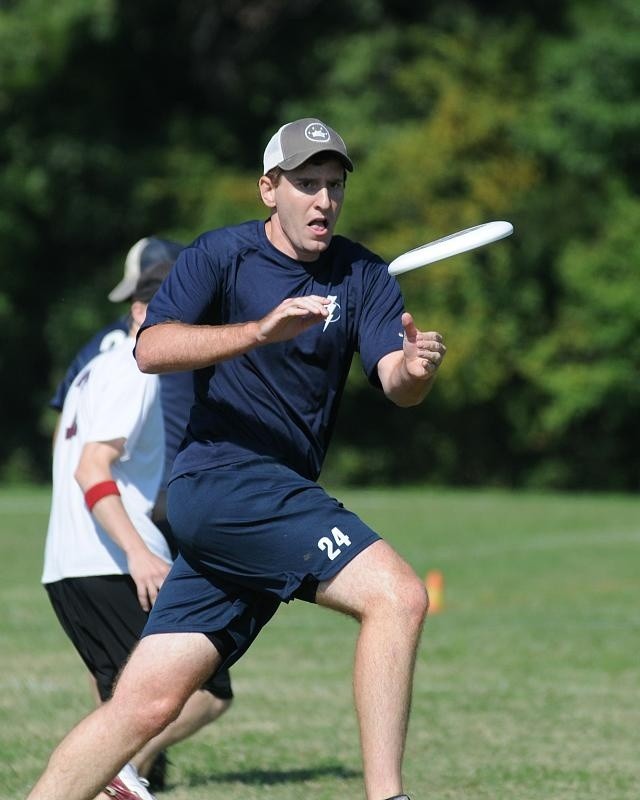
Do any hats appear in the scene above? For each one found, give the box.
[263,119,355,179]
[109,235,188,303]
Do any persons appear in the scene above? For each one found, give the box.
[40,238,231,800]
[26,118,447,800]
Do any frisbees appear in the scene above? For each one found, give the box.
[387,221,514,276]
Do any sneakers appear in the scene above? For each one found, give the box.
[91,764,159,800]
